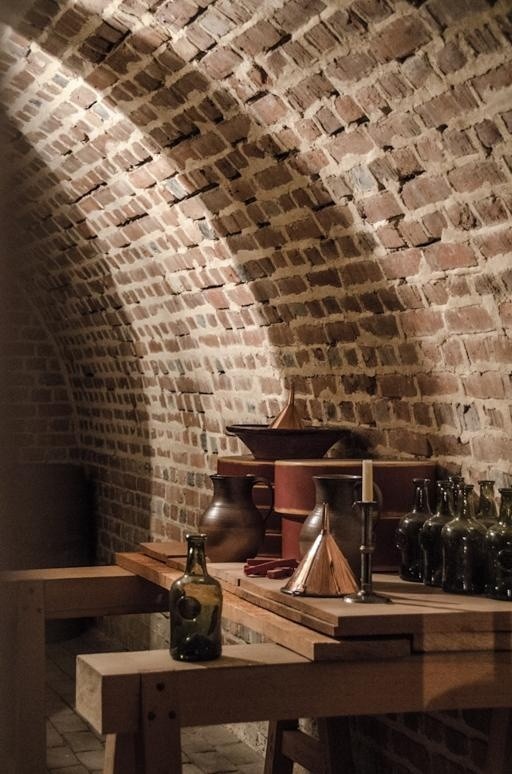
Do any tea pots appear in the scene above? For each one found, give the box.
[197,471,275,562]
[296,473,384,572]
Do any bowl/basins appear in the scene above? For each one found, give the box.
[225,421,351,461]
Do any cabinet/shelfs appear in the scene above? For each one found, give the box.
[1,540,511,774]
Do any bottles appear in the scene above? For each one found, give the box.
[392,474,512,603]
[169,532,224,663]
